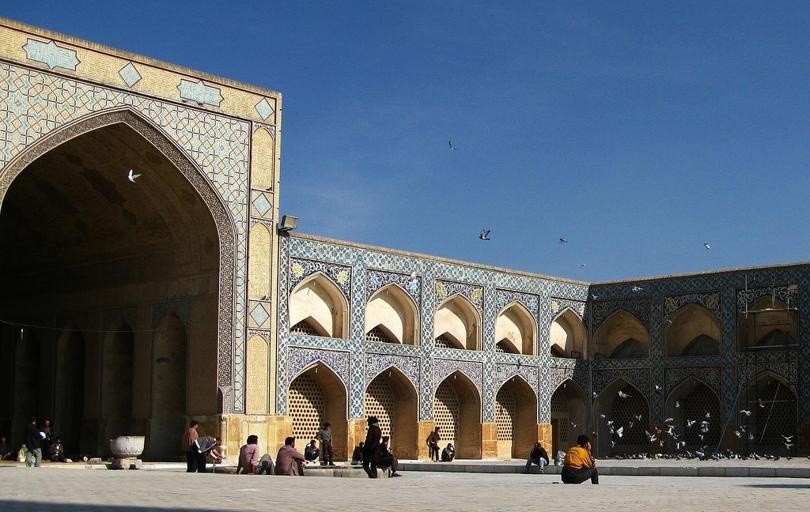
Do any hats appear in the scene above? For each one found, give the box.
[367,417,378,422]
[382,436,389,442]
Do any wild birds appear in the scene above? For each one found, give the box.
[479,229,490,240]
[632,287,642,292]
[590,292,598,300]
[128,169,142,183]
[449,141,456,151]
[560,239,568,243]
[571,385,795,462]
[704,242,711,249]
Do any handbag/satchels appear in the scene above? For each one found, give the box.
[16,448,27,464]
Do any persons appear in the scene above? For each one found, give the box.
[317,422,335,466]
[304,440,319,465]
[520,442,549,475]
[375,436,402,477]
[362,417,381,478]
[231,435,275,476]
[185,420,199,473]
[0,418,66,468]
[441,443,455,462]
[560,435,599,484]
[429,427,441,462]
[276,437,305,476]
[207,447,226,460]
[192,436,223,473]
[352,442,365,465]
